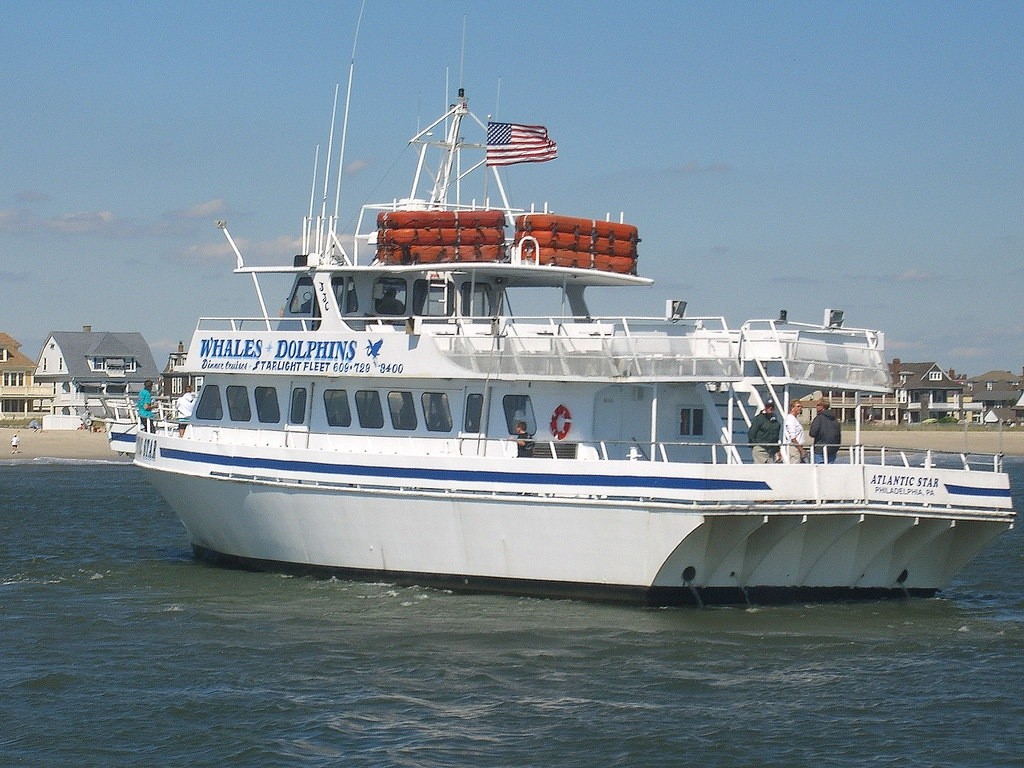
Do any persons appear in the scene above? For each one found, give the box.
[376,285,406,315]
[513,422,536,496]
[809,399,841,464]
[10,434,21,454]
[748,397,783,464]
[175,386,198,438]
[280,297,299,318]
[137,380,159,434]
[783,398,807,464]
[300,292,312,313]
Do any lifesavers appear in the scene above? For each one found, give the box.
[548,404,573,441]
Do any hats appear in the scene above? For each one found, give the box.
[14,434,17,437]
[765,399,775,406]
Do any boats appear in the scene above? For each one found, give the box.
[94,395,179,460]
[134,0,1016,604]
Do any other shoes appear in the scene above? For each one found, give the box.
[12,451,17,454]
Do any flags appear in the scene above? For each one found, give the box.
[486,121,558,167]
